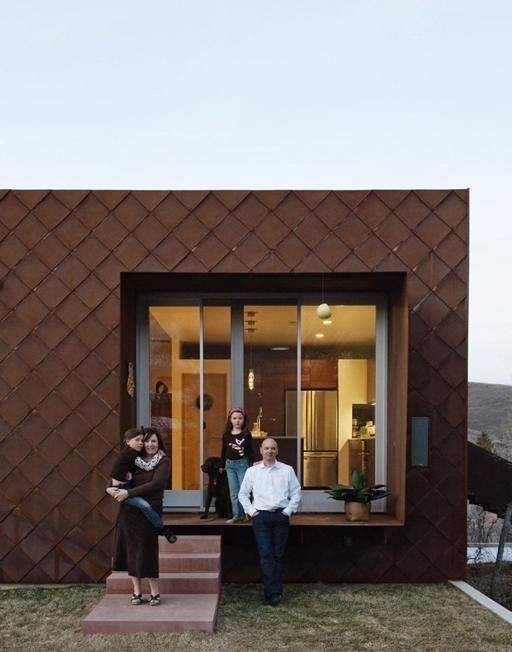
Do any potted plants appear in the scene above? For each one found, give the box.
[327,470,388,522]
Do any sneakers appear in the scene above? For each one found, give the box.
[131,592,160,606]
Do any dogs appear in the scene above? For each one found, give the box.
[200,457,233,519]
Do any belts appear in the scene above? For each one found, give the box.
[257,509,281,513]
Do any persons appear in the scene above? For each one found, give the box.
[104,427,179,605]
[219,407,252,523]
[238,438,302,606]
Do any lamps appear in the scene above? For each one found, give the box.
[245,309,256,390]
[316,277,331,320]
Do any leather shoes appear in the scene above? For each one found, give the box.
[158,525,177,544]
[264,593,282,605]
